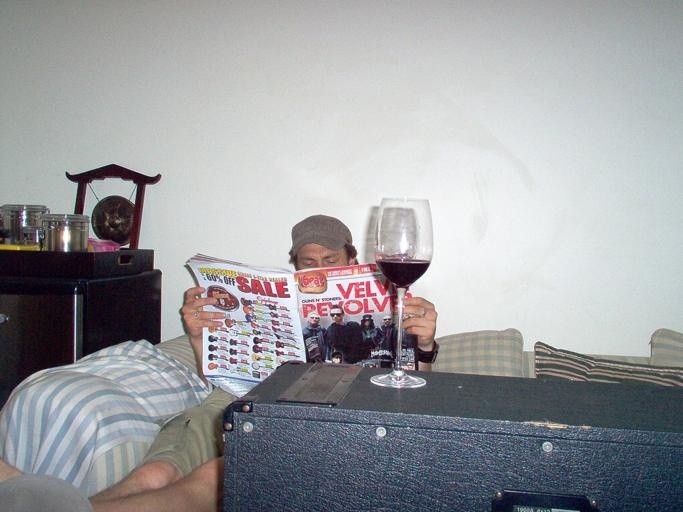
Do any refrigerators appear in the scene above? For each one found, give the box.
[0,266,162,409]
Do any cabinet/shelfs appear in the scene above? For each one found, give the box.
[3,160,162,412]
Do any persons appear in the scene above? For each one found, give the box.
[301,311,329,364]
[324,303,362,364]
[394,312,415,370]
[0,460,94,511]
[380,315,397,368]
[358,314,387,355]
[84,216,437,512]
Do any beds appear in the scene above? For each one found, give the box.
[2,330,214,500]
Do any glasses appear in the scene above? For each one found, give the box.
[310,313,392,321]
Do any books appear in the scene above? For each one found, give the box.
[183,252,417,400]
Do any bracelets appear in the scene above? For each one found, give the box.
[415,339,439,364]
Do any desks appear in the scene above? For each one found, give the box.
[221,359,683,508]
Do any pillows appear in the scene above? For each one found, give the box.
[433,330,682,386]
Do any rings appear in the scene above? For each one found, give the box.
[193,311,200,320]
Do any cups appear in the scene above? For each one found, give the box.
[370,195,435,391]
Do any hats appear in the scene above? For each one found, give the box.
[286,214,358,265]
